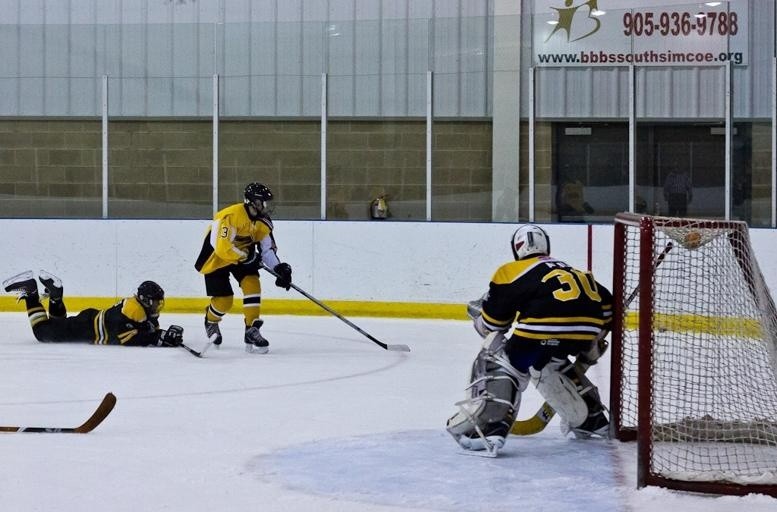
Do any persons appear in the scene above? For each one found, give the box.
[662,161,694,218]
[554,162,586,221]
[444,225,613,452]
[635,196,650,215]
[193,182,293,348]
[1,268,184,349]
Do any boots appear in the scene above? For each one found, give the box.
[4,278,40,303]
[38,276,64,300]
[205,306,222,345]
[244,317,269,347]
[573,384,610,436]
[460,369,520,449]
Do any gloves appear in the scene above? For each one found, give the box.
[161,324,184,348]
[273,262,292,291]
[241,250,263,269]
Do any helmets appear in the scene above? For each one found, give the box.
[137,281,165,308]
[511,222,551,261]
[243,182,273,205]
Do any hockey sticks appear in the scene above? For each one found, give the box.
[0,393,116,434]
[181,331,218,357]
[510,242,673,436]
[261,265,410,351]
